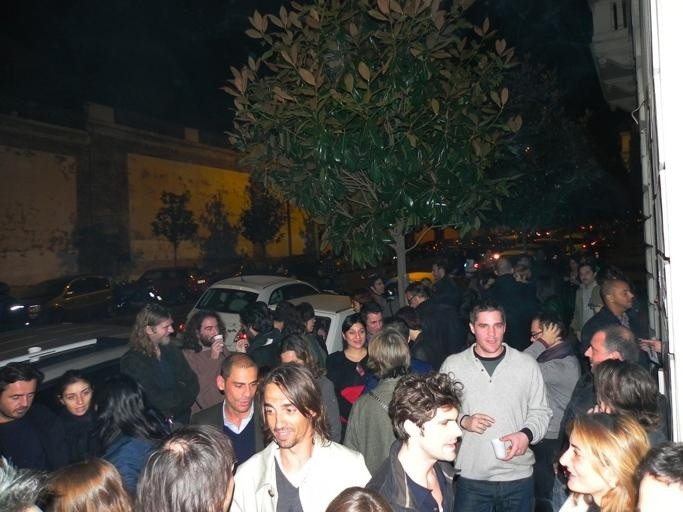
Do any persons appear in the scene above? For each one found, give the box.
[0,246,682,511]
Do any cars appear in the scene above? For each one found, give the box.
[275,229,551,276]
[266,294,355,355]
[1,275,136,324]
[183,275,342,349]
[124,267,208,304]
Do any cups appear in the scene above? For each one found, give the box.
[28,347,40,361]
[491,439,506,457]
[213,335,224,348]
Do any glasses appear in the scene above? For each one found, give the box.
[531,331,542,338]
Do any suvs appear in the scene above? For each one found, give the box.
[1,336,132,393]
